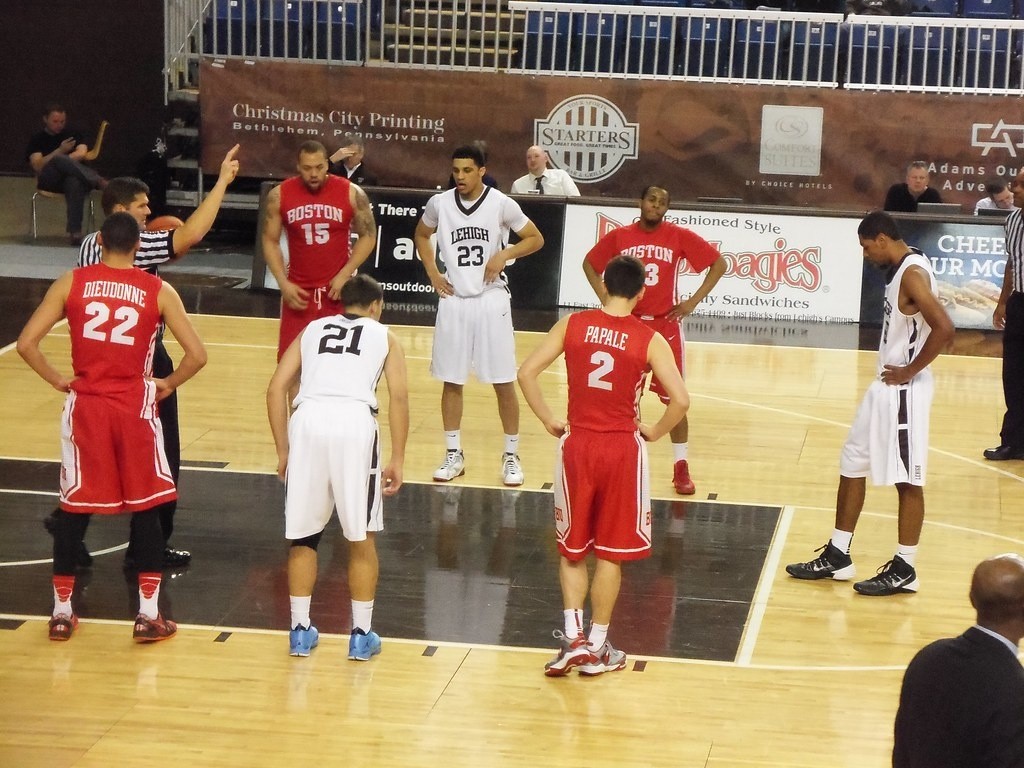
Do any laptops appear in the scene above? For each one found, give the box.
[977,208,1015,217]
[917,203,962,215]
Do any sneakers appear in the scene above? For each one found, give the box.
[785,538,856,581]
[543,629,591,678]
[672,458,696,495]
[500,451,524,486]
[432,448,466,482]
[852,554,919,596]
[132,610,178,644]
[347,626,382,661]
[288,623,319,657]
[577,638,628,676]
[47,612,79,641]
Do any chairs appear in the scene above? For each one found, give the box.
[187,0,400,91]
[516,0,1024,96]
[29,171,94,239]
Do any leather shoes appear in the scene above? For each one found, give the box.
[163,546,192,568]
[43,509,94,568]
[983,445,1024,461]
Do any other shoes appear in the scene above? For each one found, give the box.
[70,235,84,246]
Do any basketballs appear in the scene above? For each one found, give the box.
[147,215,190,264]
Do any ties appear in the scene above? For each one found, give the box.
[535,175,545,195]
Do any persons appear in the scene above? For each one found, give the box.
[415,144,545,485]
[785,211,955,595]
[973,176,1018,216]
[891,557,1024,768]
[511,146,581,196]
[17,211,209,640]
[448,140,498,190]
[982,166,1024,460]
[327,136,380,185]
[261,141,376,416]
[43,143,240,568]
[518,255,691,676]
[582,185,728,494]
[883,160,943,213]
[26,103,108,247]
[266,275,410,661]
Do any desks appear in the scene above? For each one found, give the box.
[249,181,1013,330]
